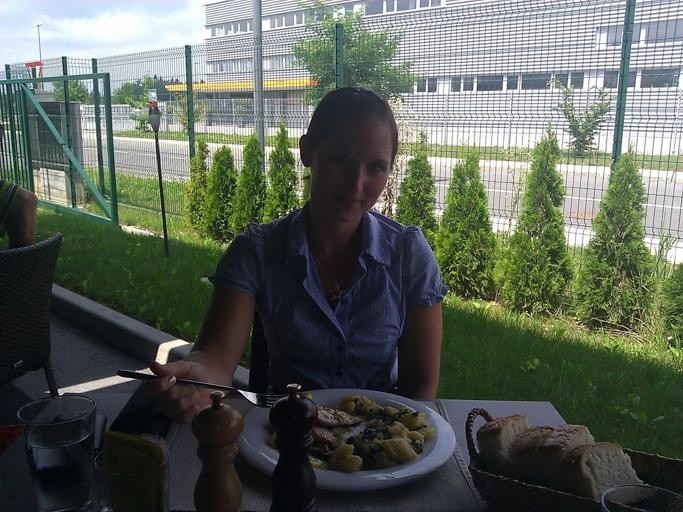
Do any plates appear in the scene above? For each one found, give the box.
[233,388,457,492]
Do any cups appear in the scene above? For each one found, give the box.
[18,395,170,512]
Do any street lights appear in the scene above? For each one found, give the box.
[33,22,44,91]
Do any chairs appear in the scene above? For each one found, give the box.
[1,231,64,398]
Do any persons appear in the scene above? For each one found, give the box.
[0,178,38,249]
[142,86,450,426]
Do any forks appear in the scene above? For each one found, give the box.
[113,369,304,409]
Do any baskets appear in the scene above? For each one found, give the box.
[465,408,682,512]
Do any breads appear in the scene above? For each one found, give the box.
[476,415,649,499]
